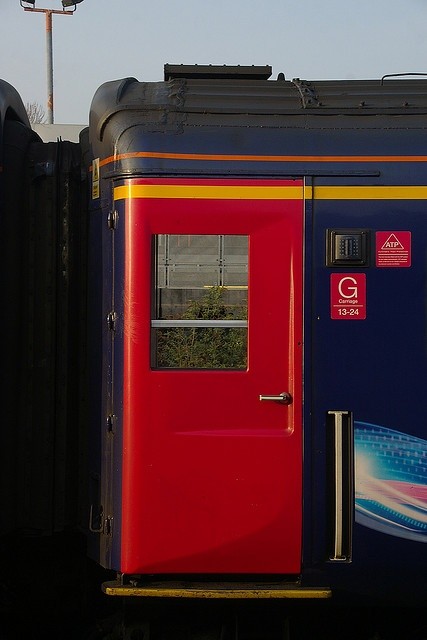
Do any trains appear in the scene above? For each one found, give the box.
[0,61,427,622]
